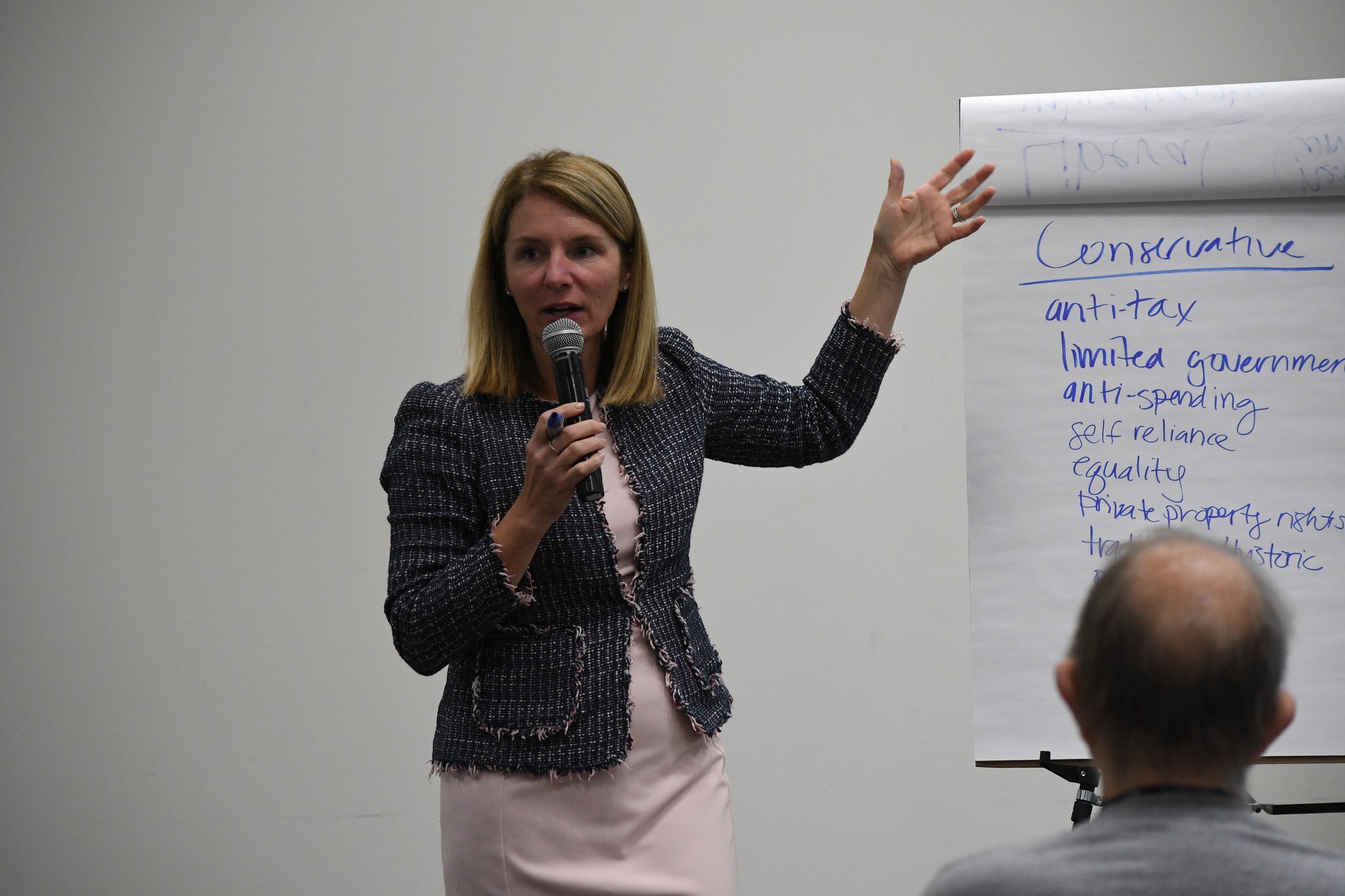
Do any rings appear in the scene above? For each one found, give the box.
[951,207,967,222]
[549,440,561,454]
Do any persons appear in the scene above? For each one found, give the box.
[924,527,1344,896]
[378,149,997,896]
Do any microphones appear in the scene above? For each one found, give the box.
[541,318,604,504]
